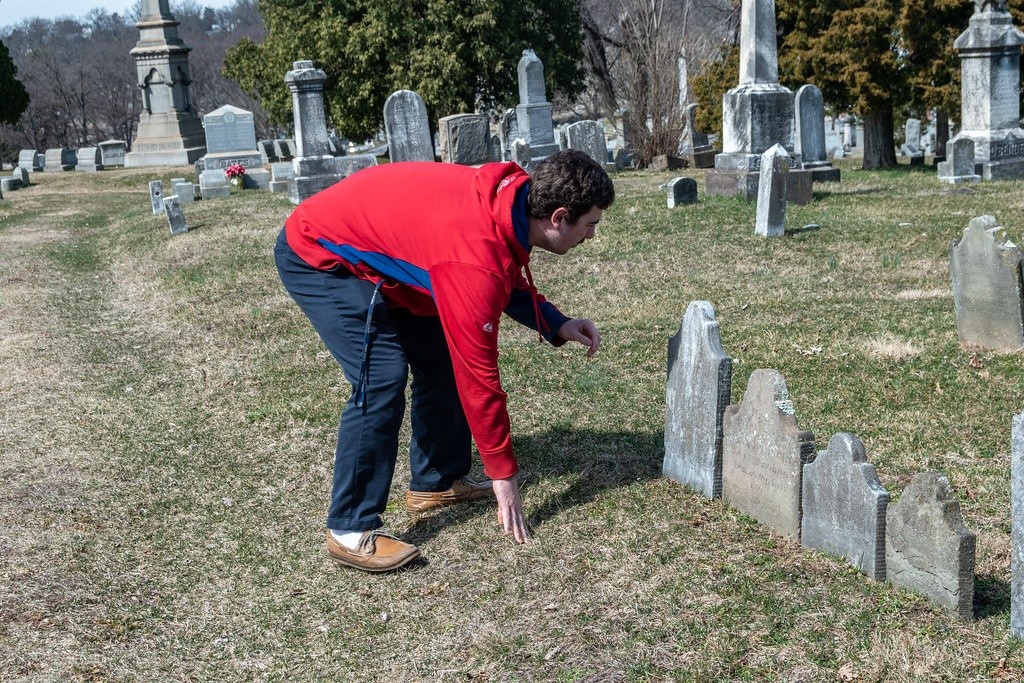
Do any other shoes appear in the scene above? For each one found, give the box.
[407,475,496,514]
[327,527,421,572]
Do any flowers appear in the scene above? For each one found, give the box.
[225,164,245,177]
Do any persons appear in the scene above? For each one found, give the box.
[274,148,615,572]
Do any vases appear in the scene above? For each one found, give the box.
[231,174,244,191]
